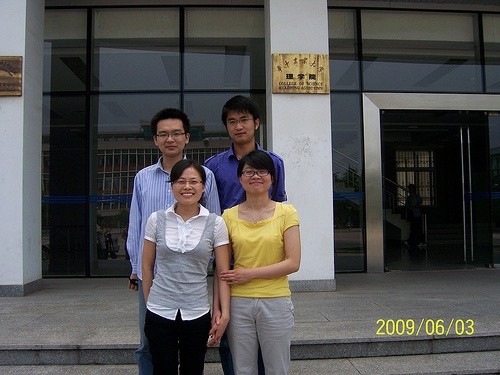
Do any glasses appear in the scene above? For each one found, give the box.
[241,169,269,177]
[172,179,204,186]
[227,119,254,125]
[156,131,186,139]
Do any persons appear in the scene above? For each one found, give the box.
[211,150,301,375]
[403,183,427,248]
[142,160,230,375]
[204,95,287,375]
[126,108,221,375]
